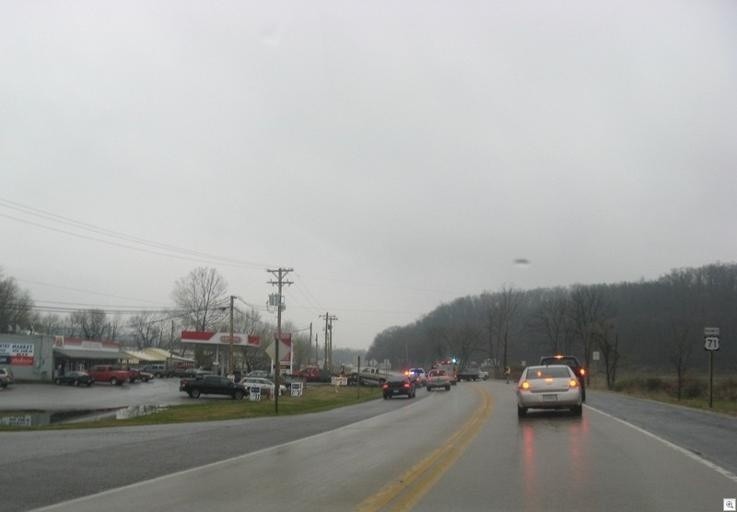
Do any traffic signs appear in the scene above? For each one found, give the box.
[704,334,721,351]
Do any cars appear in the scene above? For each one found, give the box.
[516,356,586,417]
[55,361,319,400]
[0,366,14,391]
[382,375,416,399]
[351,361,488,391]
